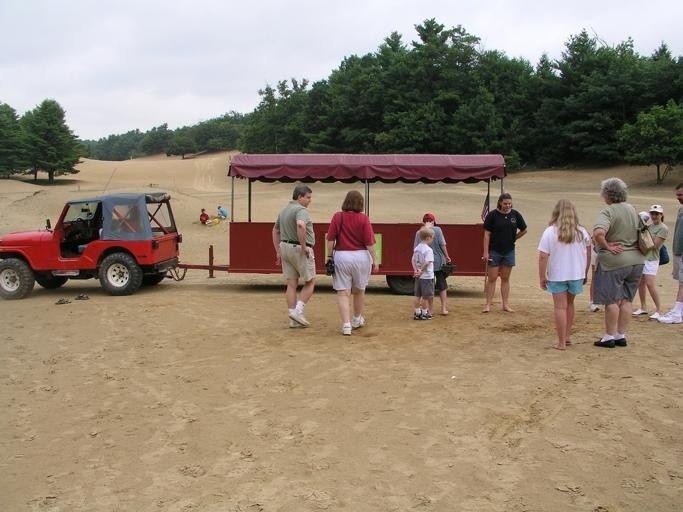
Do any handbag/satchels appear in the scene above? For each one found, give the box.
[326,258,335,277]
[659,245,670,266]
[638,224,656,256]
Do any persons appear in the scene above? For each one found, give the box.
[272,185,316,328]
[326,191,380,335]
[414,211,451,315]
[538,199,592,350]
[590,177,683,348]
[482,193,528,312]
[200,208,209,224]
[411,228,435,321]
[217,206,228,220]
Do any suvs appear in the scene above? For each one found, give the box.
[0,191,182,300]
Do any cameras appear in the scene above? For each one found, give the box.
[326,260,334,276]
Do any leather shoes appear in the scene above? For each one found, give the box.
[614,337,627,347]
[593,338,616,349]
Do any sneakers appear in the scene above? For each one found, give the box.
[656,310,683,324]
[648,311,659,319]
[288,308,310,328]
[351,315,365,328]
[342,322,352,336]
[414,310,435,321]
[632,308,648,316]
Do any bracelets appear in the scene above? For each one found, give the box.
[327,256,333,257]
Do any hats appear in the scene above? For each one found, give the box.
[423,212,437,227]
[648,204,664,214]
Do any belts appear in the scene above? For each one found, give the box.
[281,240,314,248]
